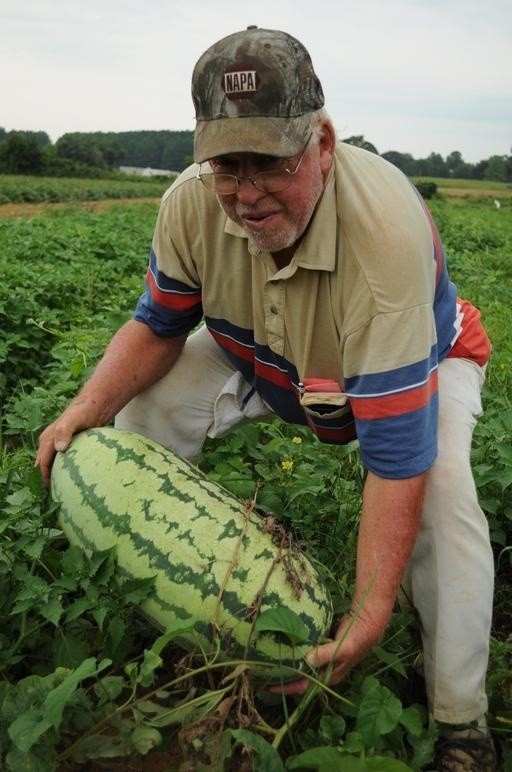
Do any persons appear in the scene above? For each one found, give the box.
[32,24,498,770]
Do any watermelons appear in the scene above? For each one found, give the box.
[49,427,335,688]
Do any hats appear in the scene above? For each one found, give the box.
[189,26,323,166]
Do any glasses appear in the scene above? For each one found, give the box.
[196,129,314,198]
